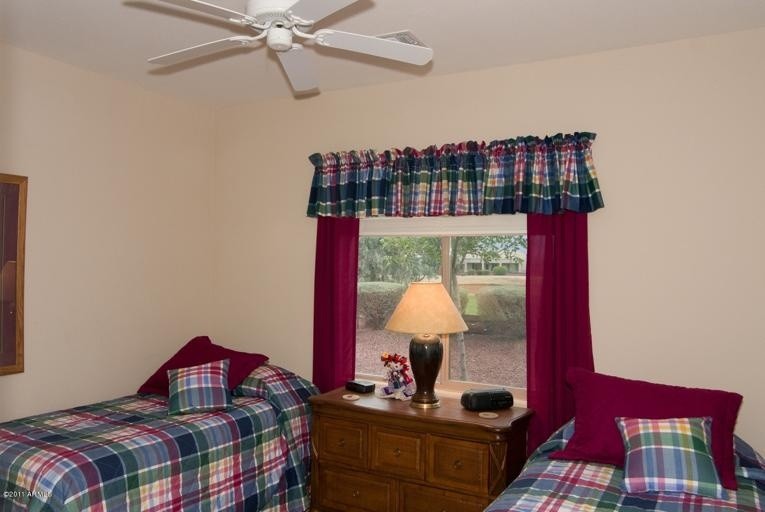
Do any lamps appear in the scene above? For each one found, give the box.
[264,27,293,51]
[383,281,468,408]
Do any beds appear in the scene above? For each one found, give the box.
[480,413,765,512]
[1,336,323,510]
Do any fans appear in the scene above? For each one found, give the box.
[146,0,434,98]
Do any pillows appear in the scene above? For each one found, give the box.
[546,368,743,491]
[136,336,269,398]
[165,357,235,416]
[615,410,733,500]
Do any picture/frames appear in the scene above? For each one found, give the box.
[0,172,29,377]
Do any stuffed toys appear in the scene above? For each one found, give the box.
[376,350,415,401]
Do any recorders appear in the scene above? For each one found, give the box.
[461,388,514,411]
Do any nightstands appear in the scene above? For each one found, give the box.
[306,384,535,512]
